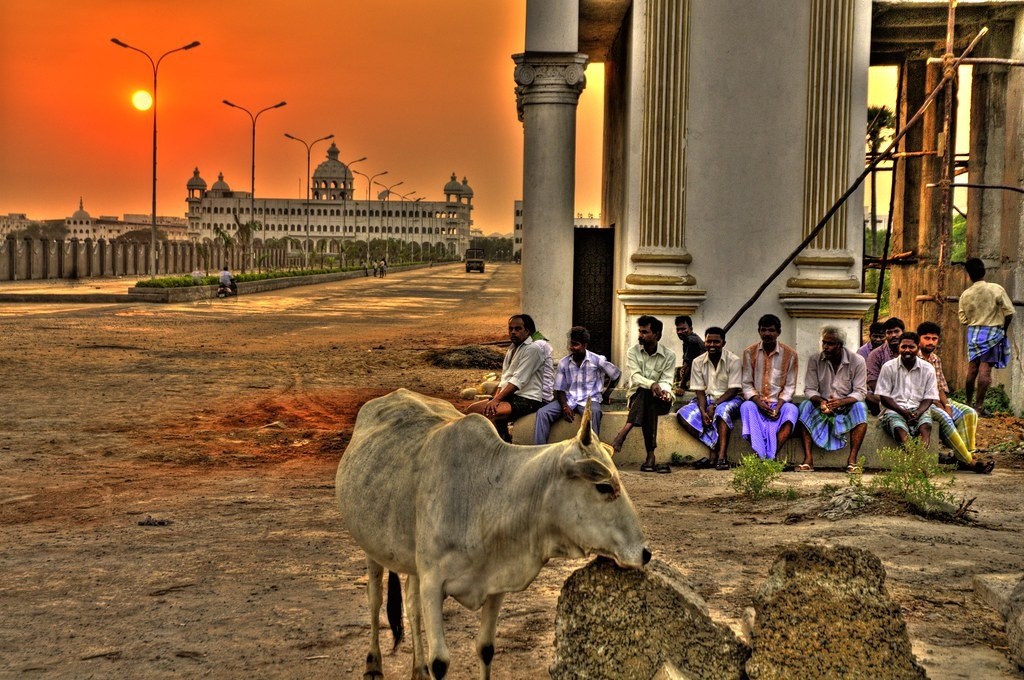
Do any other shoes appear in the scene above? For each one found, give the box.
[973,461,994,474]
[693,457,716,468]
[716,458,730,470]
[979,408,995,418]
[958,461,974,471]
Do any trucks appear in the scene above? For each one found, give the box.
[464,249,486,273]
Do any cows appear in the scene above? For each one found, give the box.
[334,389,652,680]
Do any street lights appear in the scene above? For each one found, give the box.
[283,133,335,270]
[325,155,367,268]
[373,181,404,264]
[391,191,436,262]
[222,99,287,273]
[352,169,389,266]
[109,38,201,281]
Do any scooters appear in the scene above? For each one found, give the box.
[217,278,237,298]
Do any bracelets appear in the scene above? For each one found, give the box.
[712,402,717,409]
[945,403,950,407]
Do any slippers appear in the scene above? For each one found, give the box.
[794,464,814,472]
[641,462,655,471]
[845,465,861,473]
[655,464,671,473]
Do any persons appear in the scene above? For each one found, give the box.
[379,257,388,279]
[875,331,939,477]
[465,313,554,444]
[609,315,675,467]
[533,326,622,444]
[672,317,707,396]
[792,323,869,475]
[956,256,1016,418]
[373,260,378,277]
[856,317,957,463]
[917,320,996,473]
[361,261,368,276]
[737,313,800,462]
[219,267,233,286]
[676,326,744,470]
